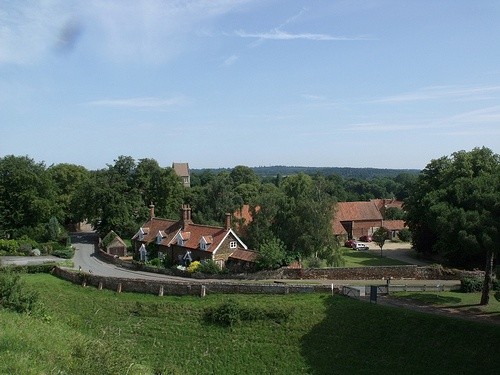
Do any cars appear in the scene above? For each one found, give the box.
[359,235,372,242]
[345,239,356,248]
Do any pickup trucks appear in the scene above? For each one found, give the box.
[353,243,369,251]
